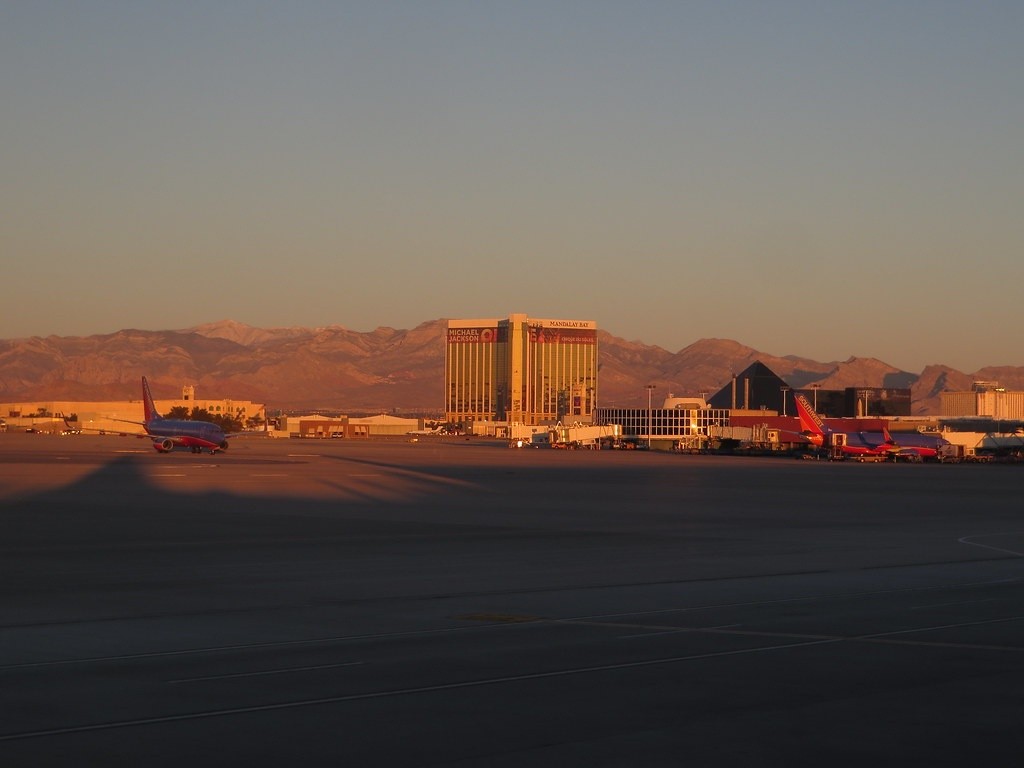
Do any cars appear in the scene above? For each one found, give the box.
[333,433,342,439]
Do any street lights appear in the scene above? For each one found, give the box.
[810,384,822,413]
[780,387,790,418]
[642,384,656,450]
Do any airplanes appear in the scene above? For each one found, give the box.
[779,392,954,463]
[78,376,273,455]
